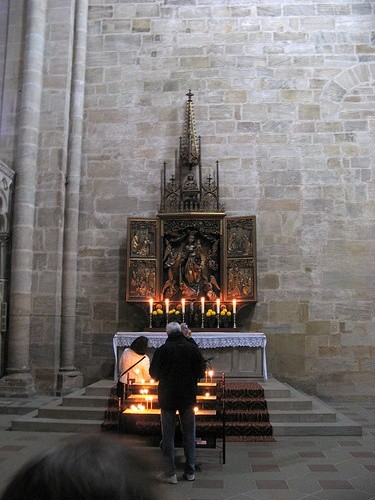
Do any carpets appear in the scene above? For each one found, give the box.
[102,380,276,442]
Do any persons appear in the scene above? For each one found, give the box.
[185,174,195,190]
[117,336,151,435]
[0,433,165,500]
[148,323,207,484]
[179,233,201,286]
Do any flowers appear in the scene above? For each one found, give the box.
[153,304,164,318]
[206,308,216,320]
[169,304,181,320]
[220,304,232,320]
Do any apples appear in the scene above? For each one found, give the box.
[153,304,163,315]
[206,309,216,316]
[169,309,180,315]
[221,309,231,316]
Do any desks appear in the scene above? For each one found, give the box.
[113,331,267,382]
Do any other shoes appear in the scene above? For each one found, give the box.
[183,472,194,481]
[155,472,177,484]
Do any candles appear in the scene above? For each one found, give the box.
[205,392,210,398]
[182,298,185,313]
[216,298,220,313]
[165,298,169,313]
[205,370,214,384]
[149,299,153,313]
[201,296,205,313]
[233,299,236,314]
[130,368,154,412]
[194,407,198,413]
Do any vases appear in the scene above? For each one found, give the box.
[153,318,163,327]
[207,320,215,328]
[223,319,230,328]
